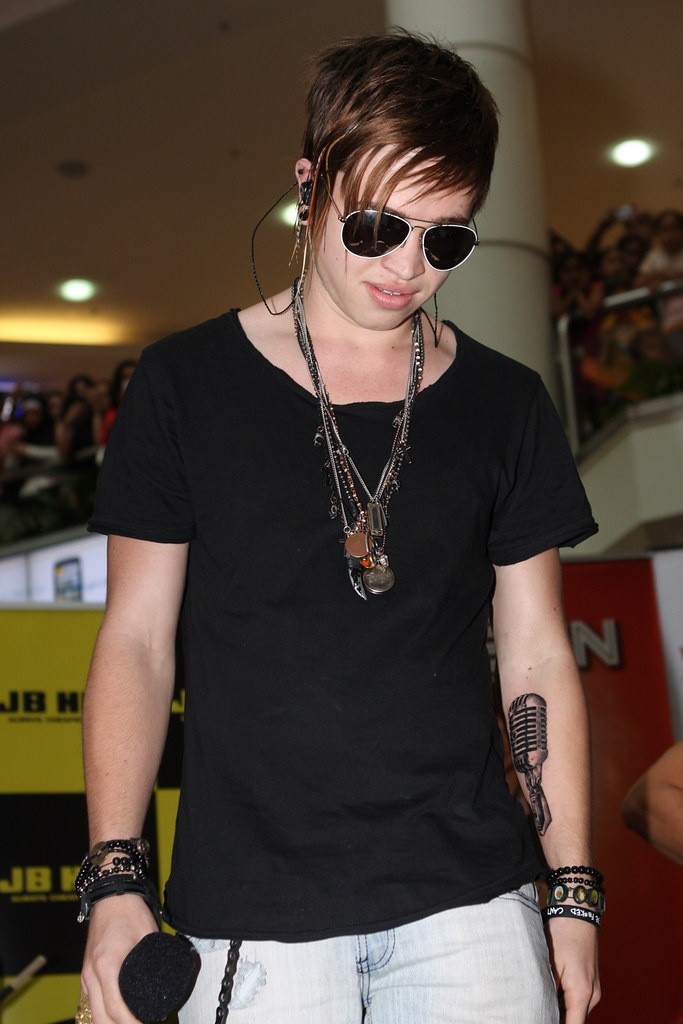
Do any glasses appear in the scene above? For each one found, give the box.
[318,173,480,273]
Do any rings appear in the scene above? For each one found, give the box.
[76,993,93,1024]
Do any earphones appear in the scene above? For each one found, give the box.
[300,181,315,220]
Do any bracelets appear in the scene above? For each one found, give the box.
[538,865,603,929]
[76,838,168,922]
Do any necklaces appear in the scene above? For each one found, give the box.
[288,272,425,601]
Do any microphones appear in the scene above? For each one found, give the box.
[119,930,201,1023]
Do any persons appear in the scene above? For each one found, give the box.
[556,197,683,440]
[621,739,683,867]
[0,355,137,513]
[74,22,606,1024]
